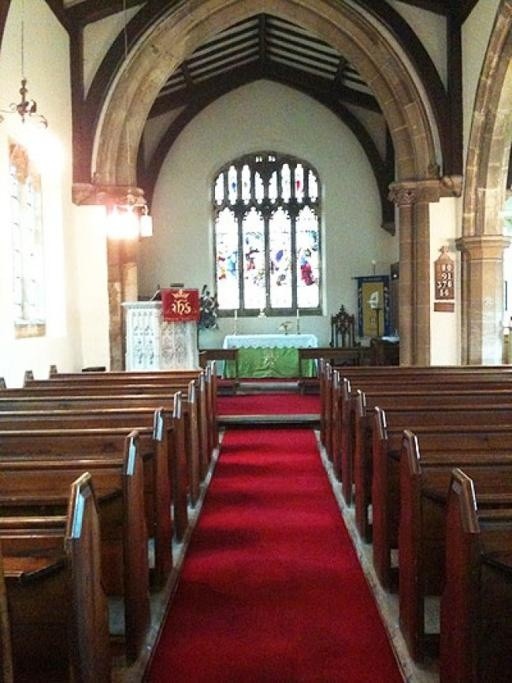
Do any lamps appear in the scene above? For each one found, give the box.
[108,0,152,241]
[0,0,48,130]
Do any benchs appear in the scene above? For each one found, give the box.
[1,361,220,682]
[319,355,512,682]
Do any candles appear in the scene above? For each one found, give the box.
[235,309,237,318]
[297,308,300,318]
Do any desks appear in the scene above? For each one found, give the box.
[222,333,319,379]
